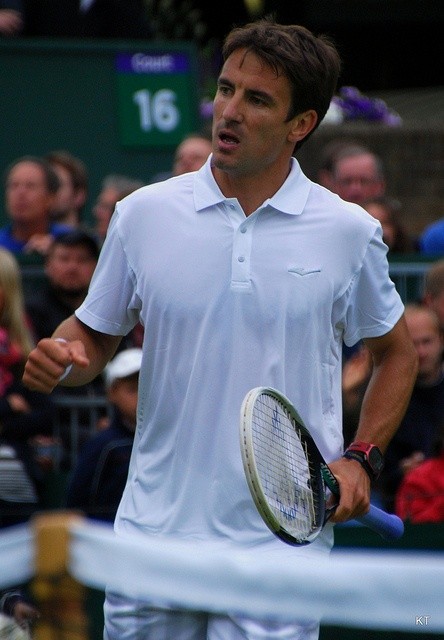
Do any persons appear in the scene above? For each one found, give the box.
[19,13,421,639]
[325,143,393,205]
[2,587,41,622]
[94,172,143,238]
[172,132,215,184]
[70,350,141,517]
[361,200,403,251]
[7,224,118,461]
[416,261,443,320]
[389,304,444,472]
[1,158,71,270]
[43,151,91,240]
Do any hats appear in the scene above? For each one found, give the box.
[105,348,144,387]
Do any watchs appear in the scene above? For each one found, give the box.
[345,441,385,482]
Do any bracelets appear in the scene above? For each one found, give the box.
[52,335,74,395]
